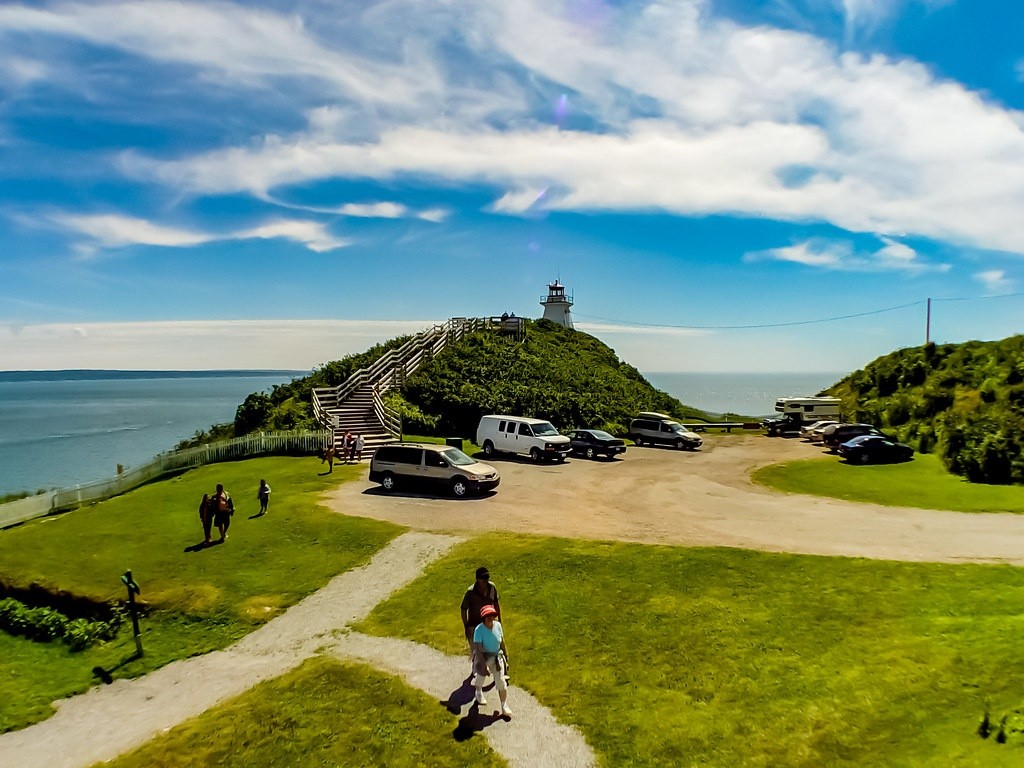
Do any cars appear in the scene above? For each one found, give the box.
[563,430,626,460]
[837,436,915,464]
[798,421,842,442]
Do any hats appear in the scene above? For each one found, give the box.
[482,605,498,618]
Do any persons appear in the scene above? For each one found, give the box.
[323,430,365,474]
[502,310,516,322]
[257,478,271,514]
[461,567,512,717]
[199,485,235,544]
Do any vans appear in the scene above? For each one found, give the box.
[477,415,573,465]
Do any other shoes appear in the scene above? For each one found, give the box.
[504,674,511,680]
[472,664,478,676]
[475,690,487,705]
[502,704,513,715]
[265,510,267,514]
[206,539,209,544]
[221,537,224,543]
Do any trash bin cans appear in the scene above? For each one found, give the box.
[446,438,463,451]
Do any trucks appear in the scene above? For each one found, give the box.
[761,396,842,436]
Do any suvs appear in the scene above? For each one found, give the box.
[368,441,500,499]
[822,424,899,451]
[625,412,703,450]
